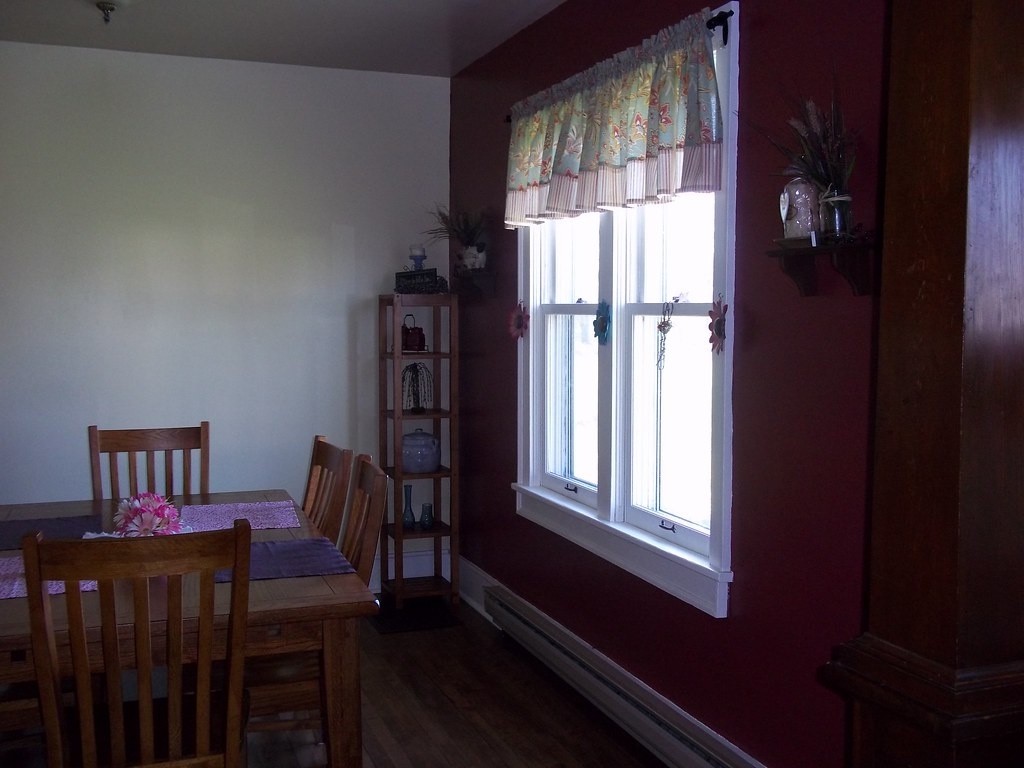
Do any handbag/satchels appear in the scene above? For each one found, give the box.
[401,314,426,351]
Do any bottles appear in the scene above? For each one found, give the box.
[402,485,415,530]
[420,503,434,530]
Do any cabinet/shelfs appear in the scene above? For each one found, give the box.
[379,294,459,610]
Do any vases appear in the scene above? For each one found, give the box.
[420,502,433,528]
[402,484,416,531]
[818,191,854,237]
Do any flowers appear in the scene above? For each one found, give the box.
[593,300,612,346]
[112,492,183,537]
[708,299,728,355]
[509,304,530,340]
[732,82,856,227]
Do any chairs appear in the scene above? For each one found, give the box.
[88,421,210,500]
[23,518,254,768]
[188,452,389,768]
[301,435,354,547]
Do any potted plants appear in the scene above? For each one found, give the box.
[419,201,498,268]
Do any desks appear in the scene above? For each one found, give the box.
[0,488,382,768]
